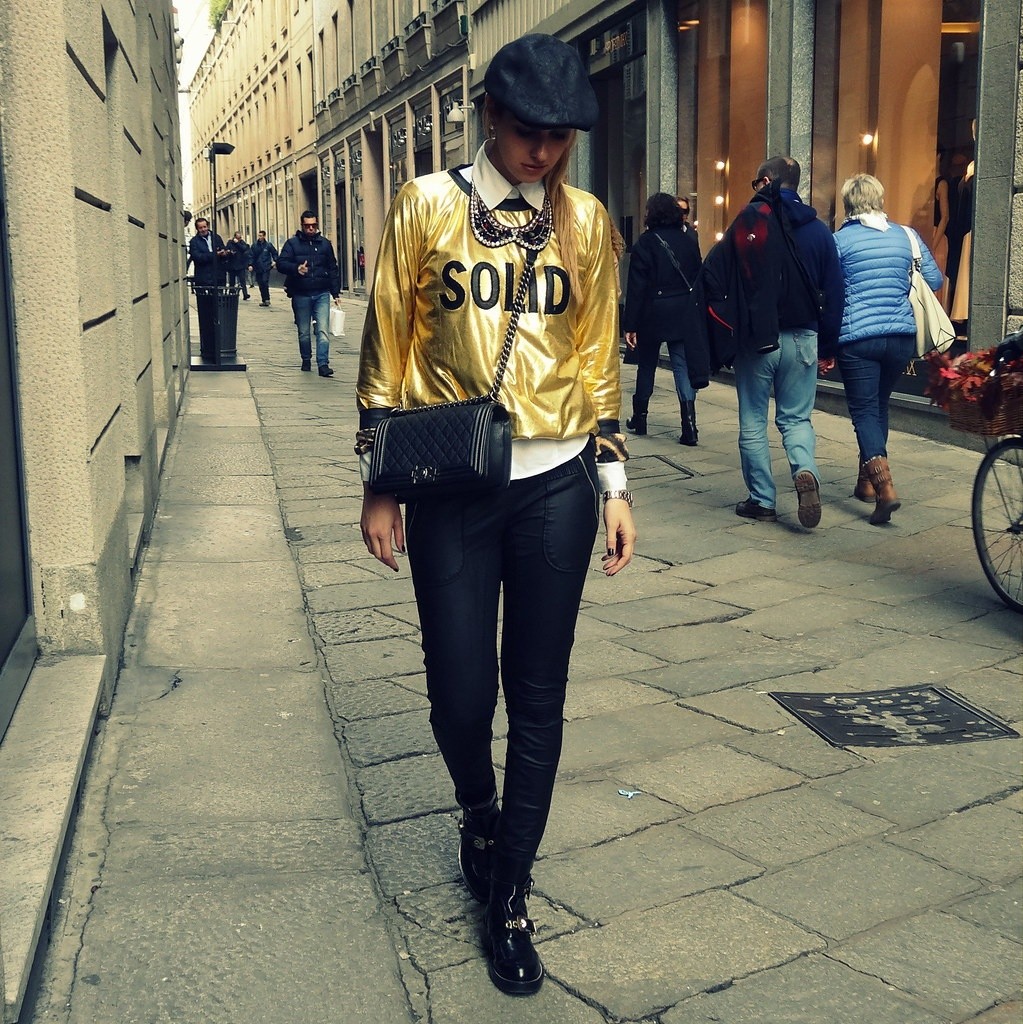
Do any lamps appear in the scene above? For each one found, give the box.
[446,100,475,123]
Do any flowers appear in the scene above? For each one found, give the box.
[926,346,1009,406]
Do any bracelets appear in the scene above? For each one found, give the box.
[603,491,633,510]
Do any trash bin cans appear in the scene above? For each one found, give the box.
[190,286,243,359]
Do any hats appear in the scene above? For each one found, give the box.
[484,33,599,132]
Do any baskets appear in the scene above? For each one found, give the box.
[943,361,1023,438]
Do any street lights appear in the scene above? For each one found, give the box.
[190,142,247,371]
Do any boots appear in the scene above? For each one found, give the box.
[312,320,318,335]
[484,872,545,994]
[452,786,501,902]
[863,454,901,525]
[855,451,877,503]
[680,400,698,445]
[627,395,649,435]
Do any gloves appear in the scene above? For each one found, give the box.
[995,327,1023,368]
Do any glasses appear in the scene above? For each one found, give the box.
[301,223,318,229]
[681,208,689,214]
[752,177,772,192]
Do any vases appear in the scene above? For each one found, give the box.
[946,370,1023,437]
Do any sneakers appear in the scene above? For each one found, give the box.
[736,497,777,522]
[796,470,821,528]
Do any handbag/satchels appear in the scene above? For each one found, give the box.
[368,394,513,504]
[900,224,956,361]
[329,300,345,337]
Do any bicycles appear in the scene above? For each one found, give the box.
[945,351,1023,612]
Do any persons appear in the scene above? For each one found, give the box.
[934,149,950,276]
[817,174,942,524]
[189,218,228,291]
[623,190,702,444]
[682,157,845,529]
[357,246,365,285]
[354,33,636,996]
[250,231,278,306]
[276,210,342,376]
[225,231,253,300]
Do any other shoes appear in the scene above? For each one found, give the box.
[244,294,250,300]
[260,300,270,306]
[319,365,333,376]
[249,284,254,288]
[301,359,310,371]
